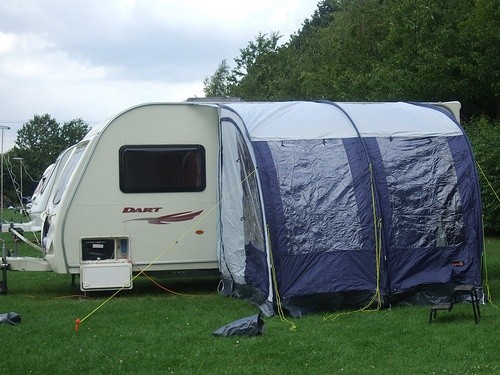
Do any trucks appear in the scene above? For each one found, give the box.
[28,157,59,215]
[30,164,54,201]
[4,95,464,295]
